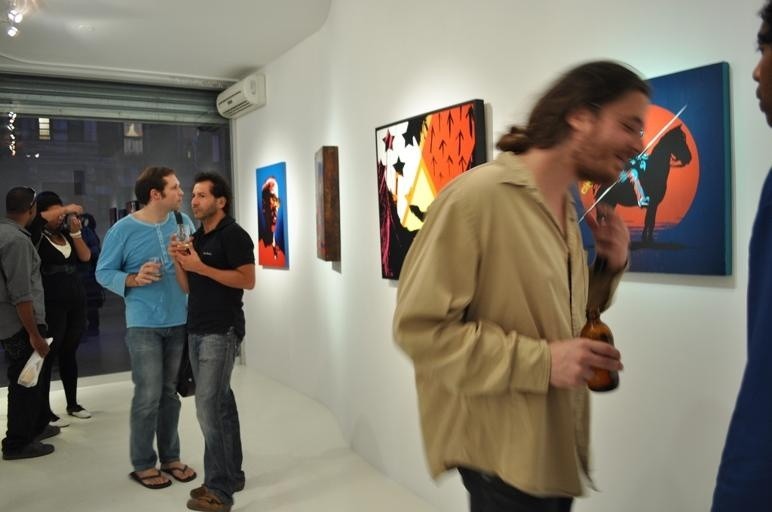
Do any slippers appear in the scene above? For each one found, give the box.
[160,462,196,482]
[129,467,173,489]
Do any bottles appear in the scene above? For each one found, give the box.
[581,305,620,392]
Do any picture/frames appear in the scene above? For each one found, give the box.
[374,98,487,280]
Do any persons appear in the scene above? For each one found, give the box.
[392,62,649,512]
[27,191,91,428]
[94,165,196,489]
[167,172,255,511]
[259,176,285,266]
[64,213,101,336]
[709,0,772,512]
[0,187,61,460]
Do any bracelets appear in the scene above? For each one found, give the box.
[72,235,82,239]
[70,230,82,236]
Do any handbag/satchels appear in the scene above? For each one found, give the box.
[174,330,198,398]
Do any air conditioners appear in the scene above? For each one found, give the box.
[215,72,267,121]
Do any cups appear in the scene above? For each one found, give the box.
[177,223,189,255]
[150,257,165,278]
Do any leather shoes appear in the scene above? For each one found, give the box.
[67,405,91,418]
[3,444,55,460]
[36,426,60,441]
[48,416,71,427]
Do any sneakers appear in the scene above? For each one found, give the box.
[190,473,245,498]
[187,493,233,512]
